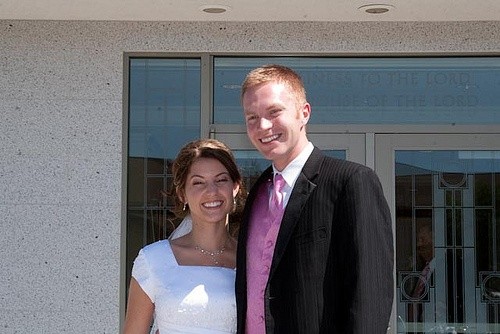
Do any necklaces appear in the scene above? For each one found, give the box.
[188,227,233,255]
[186,233,232,266]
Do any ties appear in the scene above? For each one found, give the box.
[254,173,284,329]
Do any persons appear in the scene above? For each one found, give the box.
[224,62,397,333]
[409,215,466,325]
[120,133,245,333]
